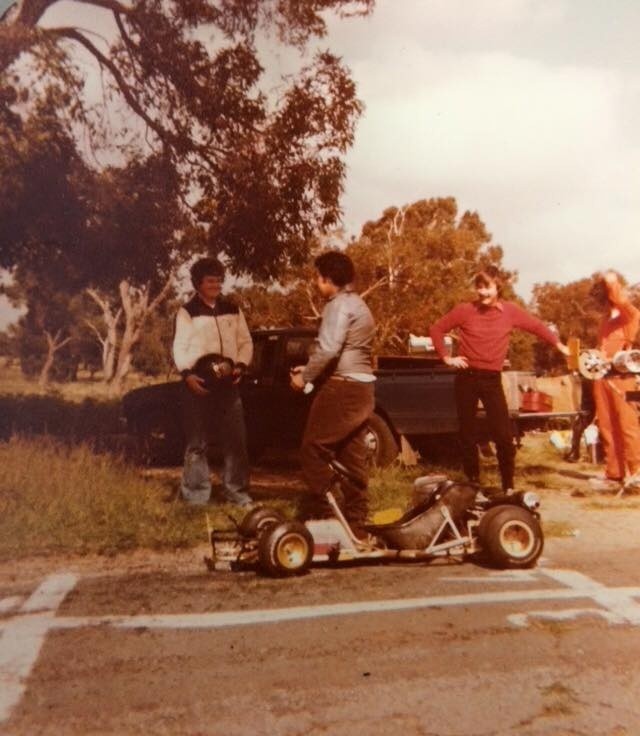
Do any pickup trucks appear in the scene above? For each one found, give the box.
[117,328,587,481]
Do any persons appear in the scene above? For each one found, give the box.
[289,250,376,543]
[565,281,640,462]
[428,265,571,495]
[576,270,640,497]
[171,257,258,508]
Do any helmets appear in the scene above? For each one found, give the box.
[191,352,236,393]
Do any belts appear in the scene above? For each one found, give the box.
[327,374,360,383]
